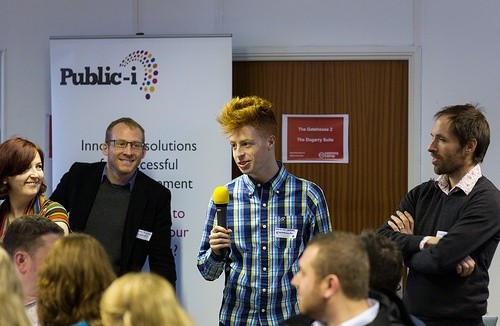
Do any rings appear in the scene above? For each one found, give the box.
[398,227,405,230]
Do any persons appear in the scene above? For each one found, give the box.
[45,117,176,292]
[278,230,424,326]
[196,97,332,326]
[375,103,500,326]
[0,138,193,326]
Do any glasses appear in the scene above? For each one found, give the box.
[106,140,145,151]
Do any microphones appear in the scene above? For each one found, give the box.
[213,185,229,257]
